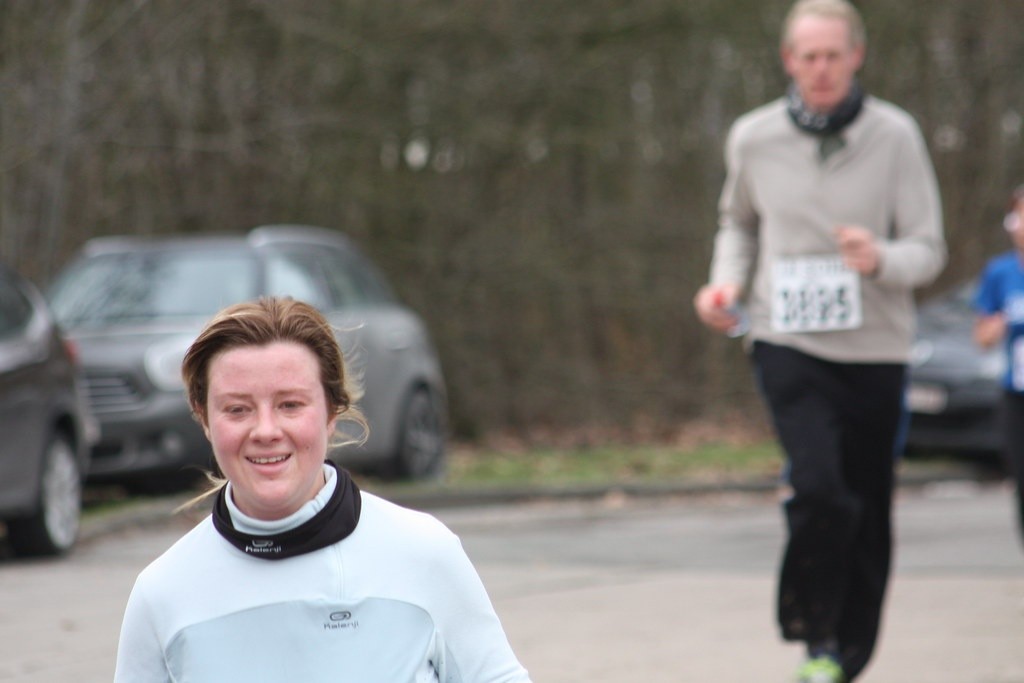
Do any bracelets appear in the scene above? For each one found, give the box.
[858,256,884,281]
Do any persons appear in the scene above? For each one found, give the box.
[114,293,536,683]
[693,0,951,683]
[967,184,1024,544]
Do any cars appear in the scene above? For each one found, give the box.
[49,221,452,494]
[901,282,1018,482]
[0,262,97,560]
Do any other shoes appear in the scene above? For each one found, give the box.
[796,654,845,683]
